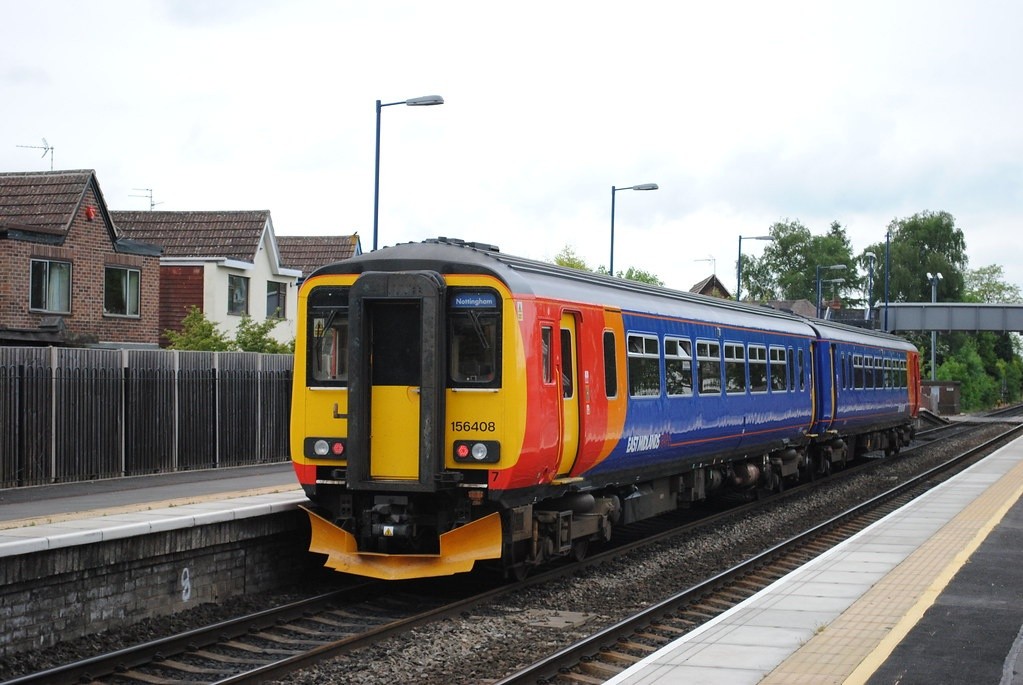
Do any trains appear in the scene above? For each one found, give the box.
[295,236,927,597]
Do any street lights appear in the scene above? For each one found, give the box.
[368,94,448,254]
[610,182,659,276]
[818,278,848,323]
[736,235,777,305]
[814,262,846,320]
